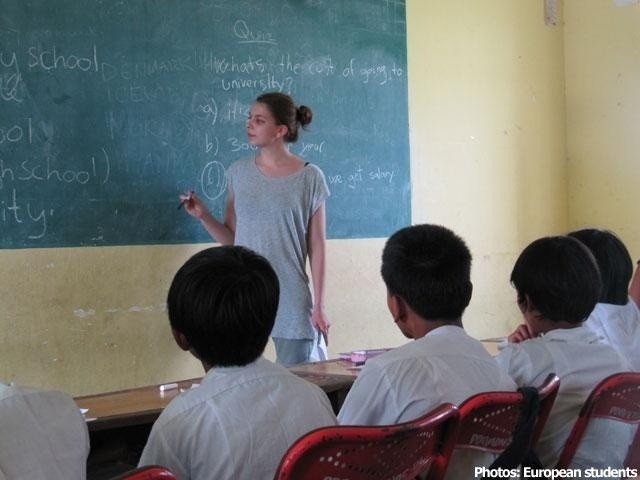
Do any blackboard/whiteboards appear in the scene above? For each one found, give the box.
[0,1,411,249]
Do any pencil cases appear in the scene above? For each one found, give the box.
[351,344,389,363]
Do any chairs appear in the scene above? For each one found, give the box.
[460,372,640,480]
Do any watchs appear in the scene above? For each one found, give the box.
[0,385,91,480]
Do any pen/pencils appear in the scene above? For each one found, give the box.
[176,191,194,210]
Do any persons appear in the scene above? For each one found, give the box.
[179,92,331,365]
[495,229,640,480]
[336,225,518,480]
[138,245,338,480]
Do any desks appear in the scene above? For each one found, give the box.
[72,335,513,480]
